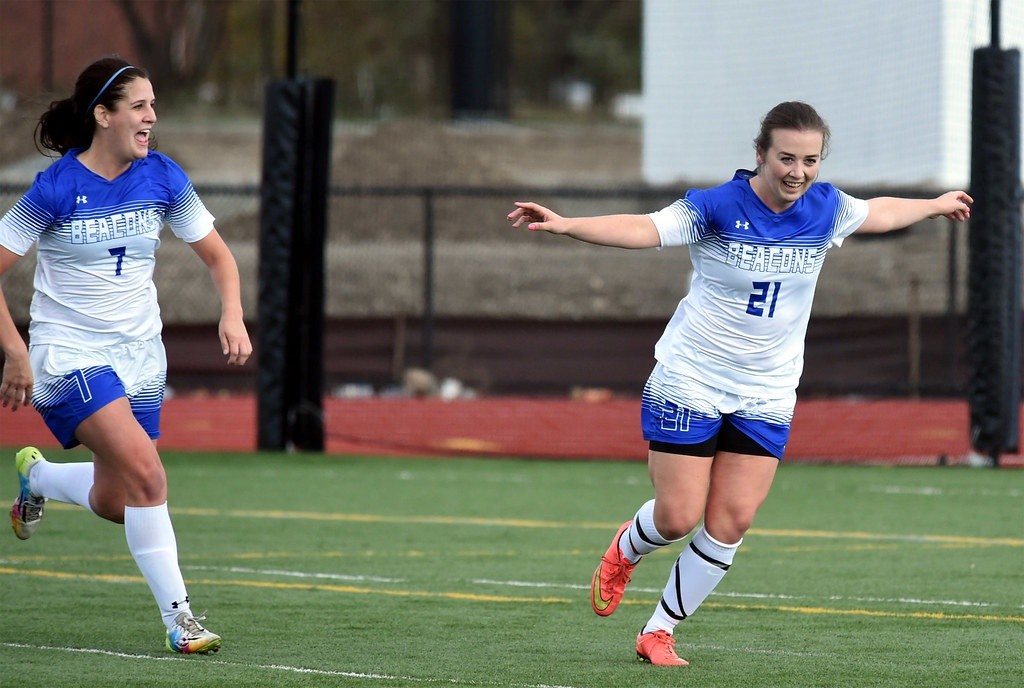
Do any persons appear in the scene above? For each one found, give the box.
[1,59,256,653]
[506,102,976,668]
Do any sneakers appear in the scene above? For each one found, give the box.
[165,612,222,654]
[635,625,689,666]
[590,520,643,618]
[9,446,49,540]
